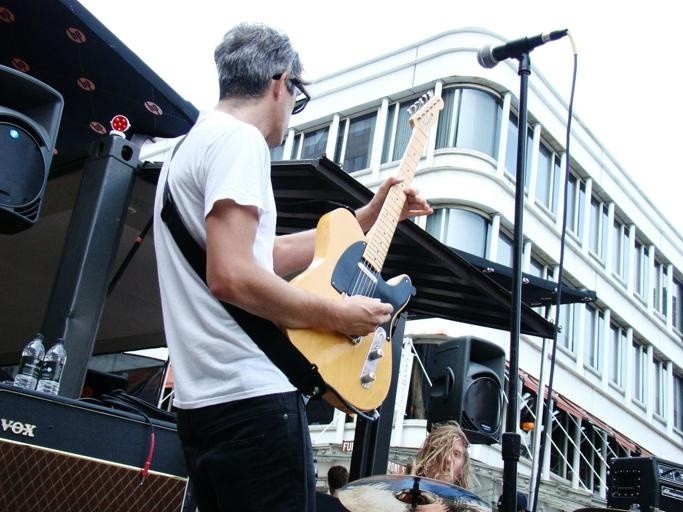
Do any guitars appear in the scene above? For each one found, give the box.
[274,90,445,416]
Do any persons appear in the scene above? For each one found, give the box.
[316,466,349,511]
[409,421,477,492]
[153,22,435,512]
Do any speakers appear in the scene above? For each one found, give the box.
[0,65,65,235]
[427,336,505,443]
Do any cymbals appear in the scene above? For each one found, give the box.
[338,475,492,512]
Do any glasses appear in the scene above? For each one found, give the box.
[272,74,311,114]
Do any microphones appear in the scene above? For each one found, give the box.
[477,29,567,68]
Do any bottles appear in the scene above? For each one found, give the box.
[35,336,67,394]
[312,459,319,481]
[12,333,45,392]
